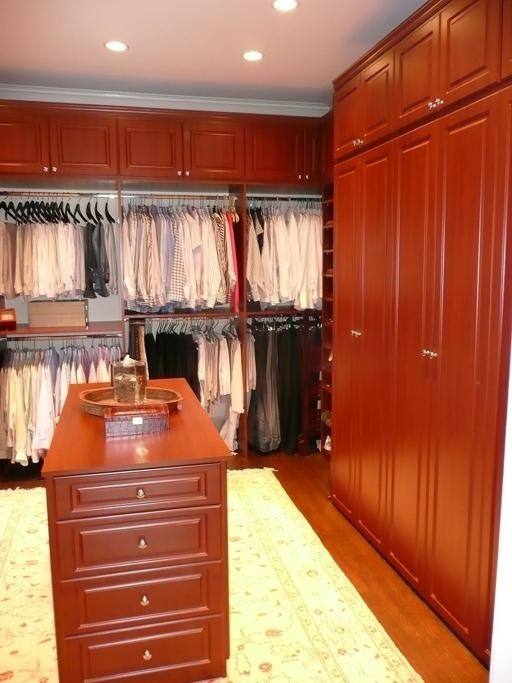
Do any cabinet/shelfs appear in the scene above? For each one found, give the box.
[396,2,512,130]
[1,98,325,186]
[329,138,396,571]
[326,29,394,163]
[398,88,511,670]
[40,377,237,682]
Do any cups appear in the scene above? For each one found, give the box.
[111,361,146,401]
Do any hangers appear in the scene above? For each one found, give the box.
[0,336,119,373]
[142,315,241,351]
[1,190,321,228]
